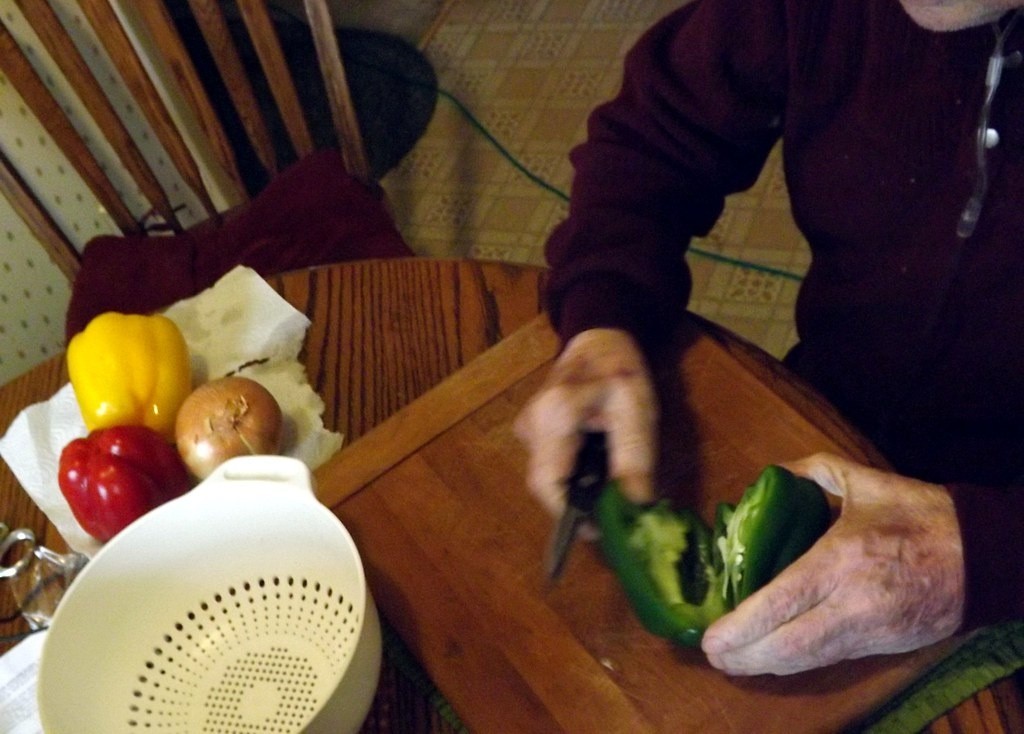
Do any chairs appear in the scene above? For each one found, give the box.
[0,2,412,343]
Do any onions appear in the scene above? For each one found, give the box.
[174,374,283,480]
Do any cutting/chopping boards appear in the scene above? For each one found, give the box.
[312,305,979,734]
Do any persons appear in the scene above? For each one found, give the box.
[505,0,1024,675]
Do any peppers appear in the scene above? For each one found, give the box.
[595,467,832,652]
[57,305,195,545]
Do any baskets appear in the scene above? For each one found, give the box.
[36,452,382,734]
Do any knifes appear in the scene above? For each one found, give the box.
[547,433,606,578]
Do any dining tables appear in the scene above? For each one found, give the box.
[0,252,1024,733]
